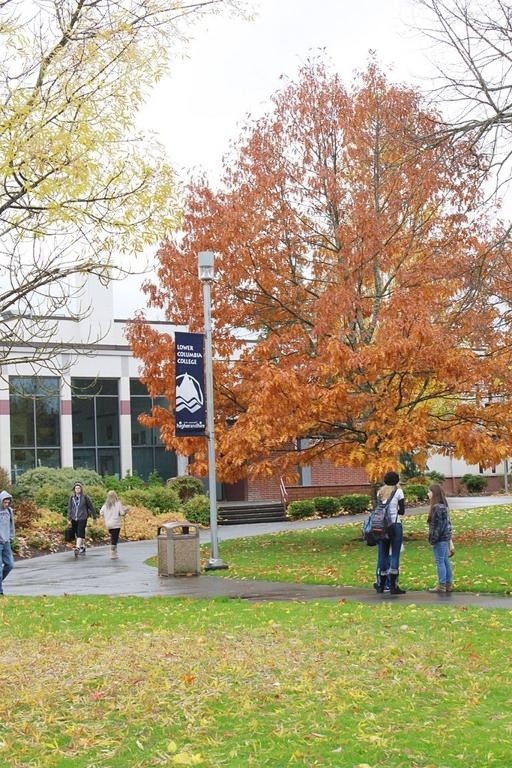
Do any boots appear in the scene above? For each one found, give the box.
[110,544,120,559]
[373,567,388,593]
[74,538,86,556]
[388,568,407,594]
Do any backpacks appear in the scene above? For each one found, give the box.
[362,505,377,547]
[369,486,400,542]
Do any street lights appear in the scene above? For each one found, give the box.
[197,250,229,570]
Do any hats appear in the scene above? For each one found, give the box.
[383,471,399,485]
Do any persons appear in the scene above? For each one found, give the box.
[65,483,97,555]
[363,472,406,594]
[99,490,129,560]
[426,485,456,593]
[0,490,15,595]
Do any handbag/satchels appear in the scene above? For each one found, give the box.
[447,537,456,557]
[65,527,75,542]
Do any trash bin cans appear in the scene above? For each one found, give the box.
[157,522,201,577]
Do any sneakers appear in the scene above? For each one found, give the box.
[428,584,453,593]
